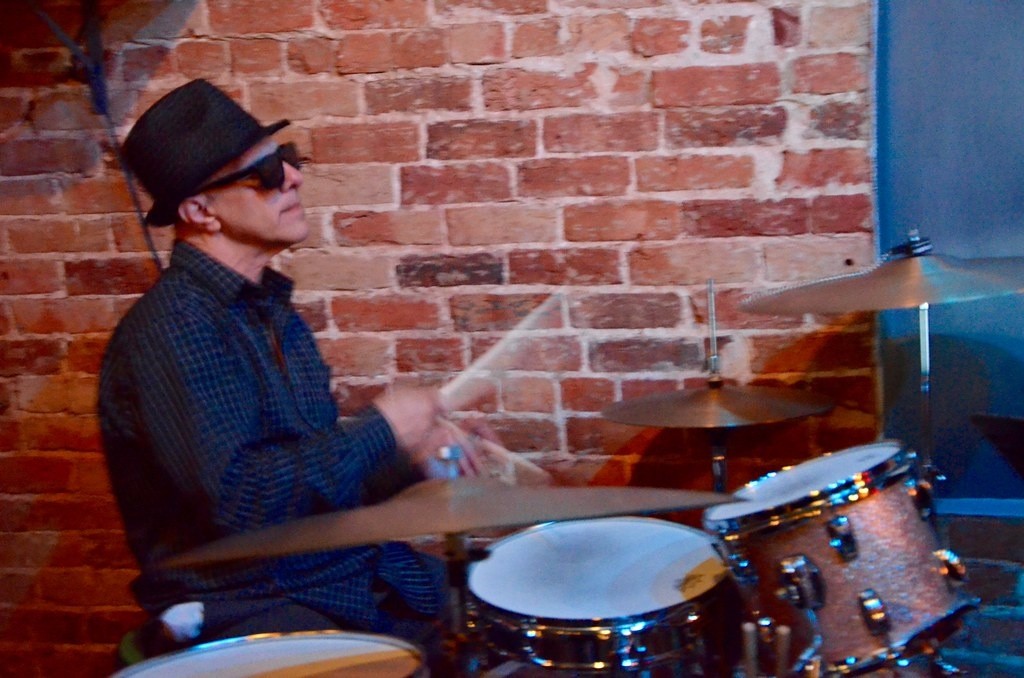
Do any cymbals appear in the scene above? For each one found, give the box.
[732,249,1024,319]
[153,472,749,573]
[601,384,833,430]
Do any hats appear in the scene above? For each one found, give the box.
[121,79,290,227]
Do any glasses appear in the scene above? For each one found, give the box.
[191,142,300,197]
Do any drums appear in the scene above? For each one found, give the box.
[462,515,734,678]
[705,435,983,677]
[113,627,432,678]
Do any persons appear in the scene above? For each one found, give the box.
[95,78,506,678]
